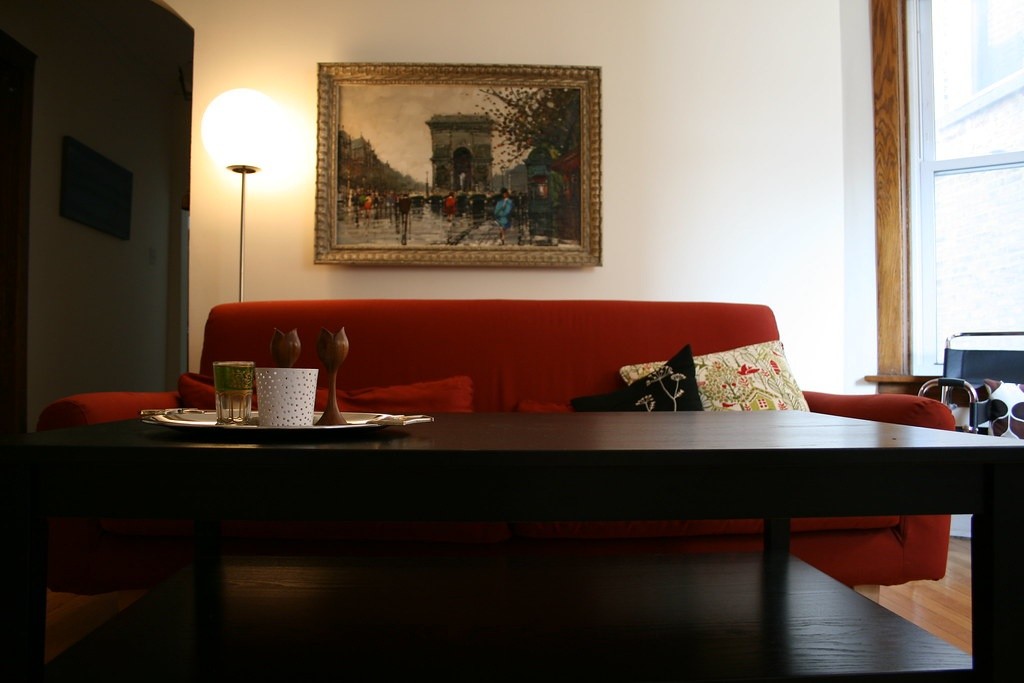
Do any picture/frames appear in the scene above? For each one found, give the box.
[313,62,602,267]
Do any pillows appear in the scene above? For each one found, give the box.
[621,342,811,414]
[177,371,474,413]
[570,341,704,414]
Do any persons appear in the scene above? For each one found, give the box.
[336,185,529,244]
[493,191,512,244]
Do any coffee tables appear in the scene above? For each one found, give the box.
[0,413,1024,683]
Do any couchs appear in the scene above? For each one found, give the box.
[36,301,955,605]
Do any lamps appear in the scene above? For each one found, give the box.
[200,87,288,303]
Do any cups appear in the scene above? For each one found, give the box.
[212,361,255,424]
